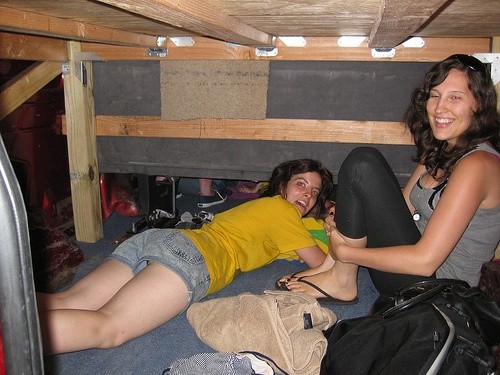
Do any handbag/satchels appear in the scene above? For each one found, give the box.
[323,278,500,375]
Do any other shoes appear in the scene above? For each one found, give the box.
[197,190,228,209]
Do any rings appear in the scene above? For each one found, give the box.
[326,232,331,237]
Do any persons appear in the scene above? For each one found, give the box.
[35,159,336,358]
[155,174,229,208]
[232,180,337,219]
[276,55,500,306]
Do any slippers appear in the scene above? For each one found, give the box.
[275,271,300,288]
[280,277,359,306]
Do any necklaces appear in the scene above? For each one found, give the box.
[413,160,445,221]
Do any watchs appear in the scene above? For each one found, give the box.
[325,200,335,211]
